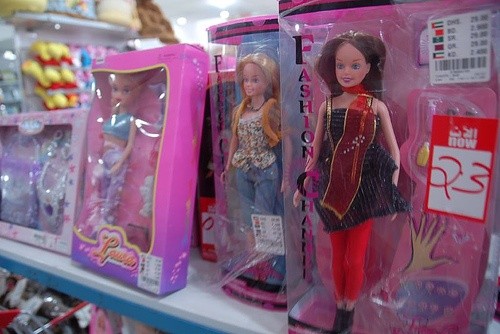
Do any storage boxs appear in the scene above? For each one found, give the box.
[71,43,208,295]
[0,108,92,255]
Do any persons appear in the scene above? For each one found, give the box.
[90,69,144,249]
[218,53,285,288]
[290,32,401,333]
